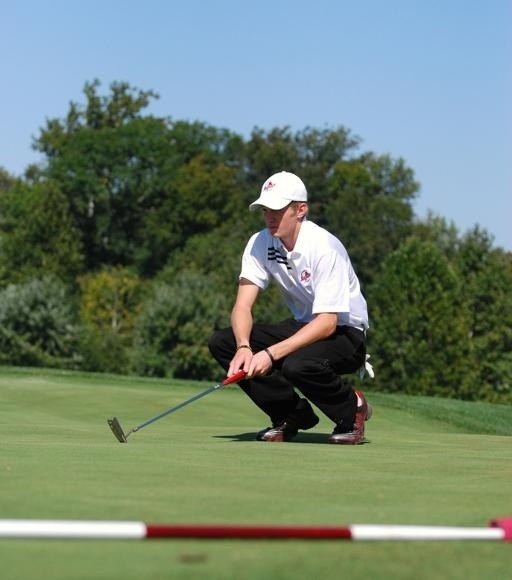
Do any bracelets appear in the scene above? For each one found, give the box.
[234,344,254,351]
[263,348,276,368]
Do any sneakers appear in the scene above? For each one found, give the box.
[256,397,320,443]
[329,385,374,446]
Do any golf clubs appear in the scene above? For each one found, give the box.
[108,364,250,445]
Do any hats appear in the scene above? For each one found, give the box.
[248,169,309,213]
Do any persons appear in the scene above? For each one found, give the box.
[207,170,375,446]
